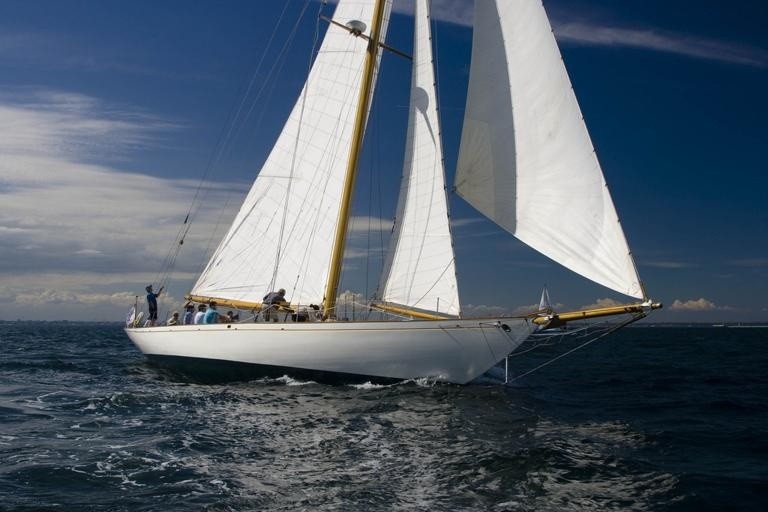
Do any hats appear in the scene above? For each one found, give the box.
[146,285,152,292]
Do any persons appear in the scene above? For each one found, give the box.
[182,305,195,325]
[292,304,320,321]
[203,301,231,324]
[193,304,207,325]
[166,312,182,325]
[224,311,238,323]
[146,285,164,327]
[261,289,289,322]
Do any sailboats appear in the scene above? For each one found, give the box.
[123,1,664,390]
